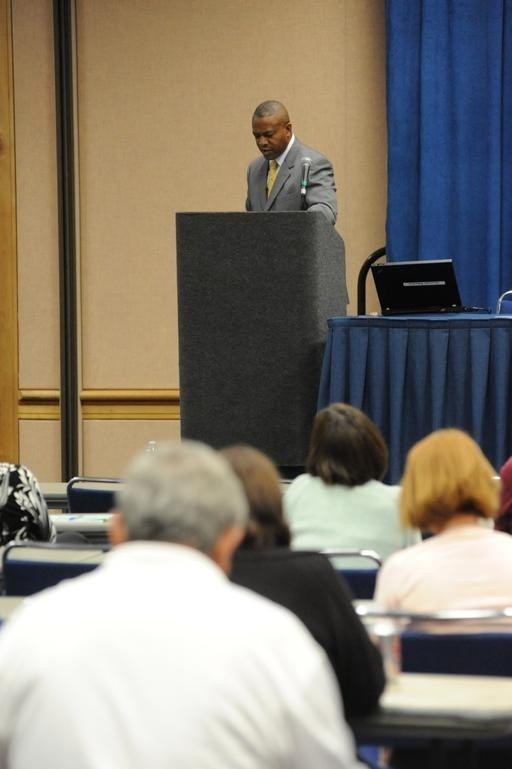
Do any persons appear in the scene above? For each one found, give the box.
[218,442,385,723]
[244,100,338,226]
[1,438,365,768]
[277,402,421,568]
[369,427,511,605]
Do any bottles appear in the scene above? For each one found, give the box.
[145,440,158,452]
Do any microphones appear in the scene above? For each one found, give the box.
[299,155,313,195]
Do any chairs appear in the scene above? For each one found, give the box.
[0,474,512,769]
[496,290,512,315]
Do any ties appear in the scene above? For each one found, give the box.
[266,159,277,199]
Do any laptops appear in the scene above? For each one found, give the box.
[370,257,492,316]
